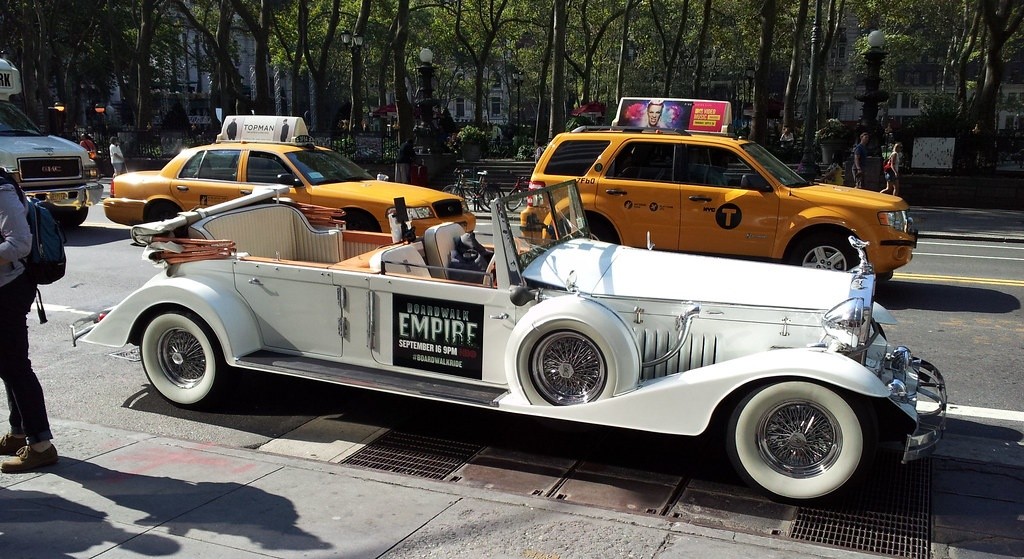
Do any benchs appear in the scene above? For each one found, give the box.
[190,168,238,181]
[189,202,343,258]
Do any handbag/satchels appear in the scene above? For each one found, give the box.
[883,159,896,174]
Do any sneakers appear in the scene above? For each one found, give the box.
[0,431,27,455]
[0,444,59,472]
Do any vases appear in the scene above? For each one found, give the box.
[822,139,843,165]
[460,142,485,162]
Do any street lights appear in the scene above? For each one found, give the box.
[512,69,524,137]
[416,48,439,152]
[94,103,105,143]
[746,66,757,140]
[854,30,890,148]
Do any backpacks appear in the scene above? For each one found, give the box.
[0,178,66,285]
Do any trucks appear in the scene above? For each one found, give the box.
[0,50,105,232]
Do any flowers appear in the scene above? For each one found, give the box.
[816,118,849,140]
[444,125,489,151]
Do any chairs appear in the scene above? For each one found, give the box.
[370,243,432,277]
[424,222,471,278]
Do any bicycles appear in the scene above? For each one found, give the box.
[442,168,506,213]
[506,167,533,211]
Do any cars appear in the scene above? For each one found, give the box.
[70,178,947,507]
[103,115,476,245]
[519,97,918,279]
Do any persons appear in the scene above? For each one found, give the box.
[0,165,59,473]
[280,119,289,142]
[227,118,237,140]
[780,128,794,147]
[851,133,869,189]
[109,137,125,178]
[117,135,141,156]
[80,133,103,181]
[395,138,418,183]
[495,123,502,149]
[534,140,545,160]
[646,101,664,127]
[880,142,903,196]
[797,146,817,181]
